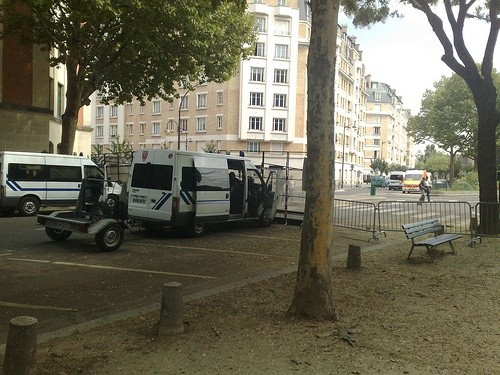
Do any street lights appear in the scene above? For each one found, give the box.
[177,78,215,151]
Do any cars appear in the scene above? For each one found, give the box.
[371,177,387,188]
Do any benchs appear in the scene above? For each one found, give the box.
[401,218,463,264]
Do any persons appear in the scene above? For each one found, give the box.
[419,176,431,203]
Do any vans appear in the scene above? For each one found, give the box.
[402,169,433,193]
[126,149,279,237]
[0,151,123,217]
[388,171,405,190]
